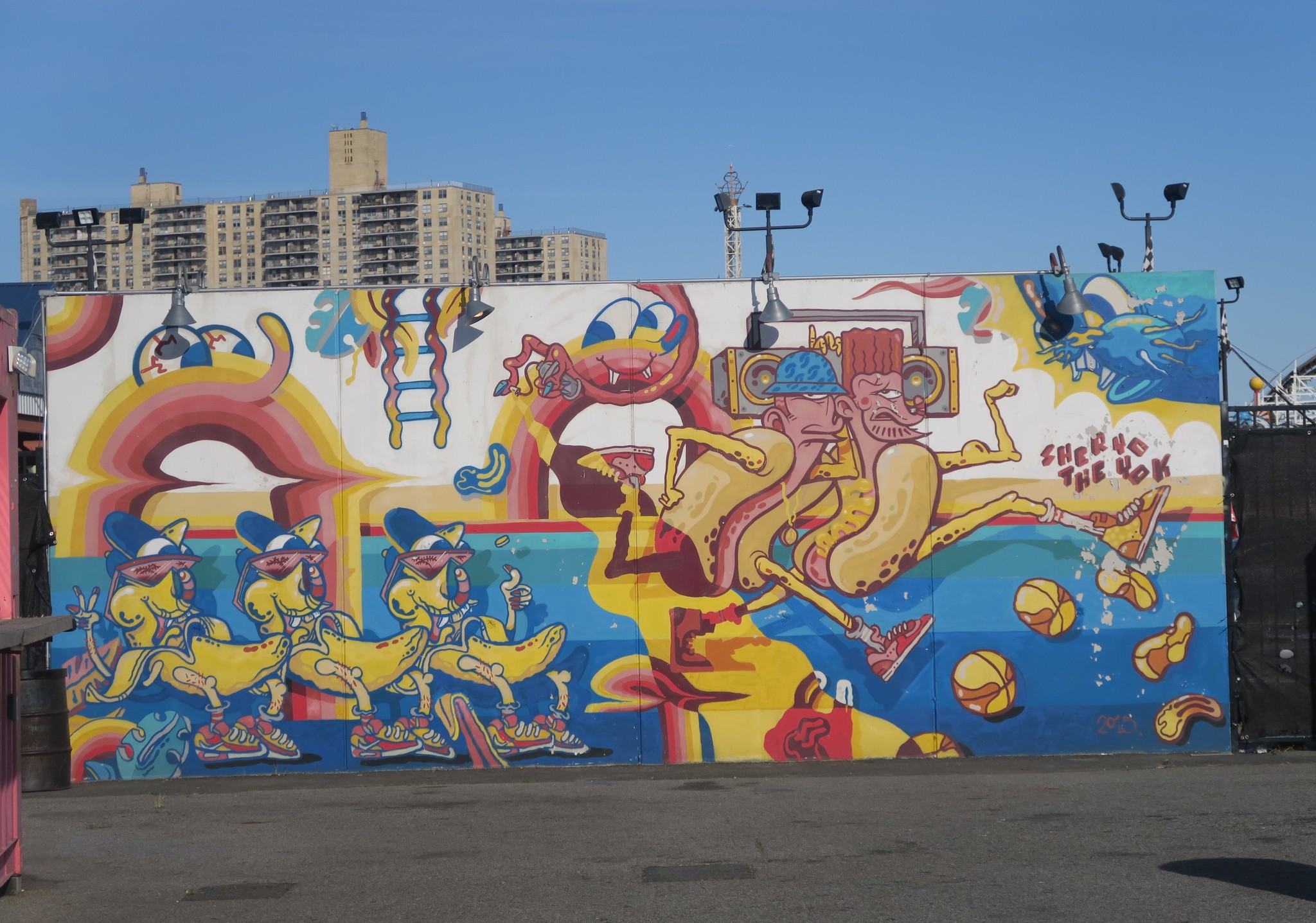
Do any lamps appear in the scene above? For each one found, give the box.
[464,254,495,328]
[160,260,198,327]
[1046,244,1093,316]
[751,255,797,324]
[8,344,38,381]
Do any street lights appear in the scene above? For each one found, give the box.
[713,187,824,278]
[34,207,145,291]
[1216,277,1244,403]
[1097,242,1124,273]
[1110,181,1191,274]
